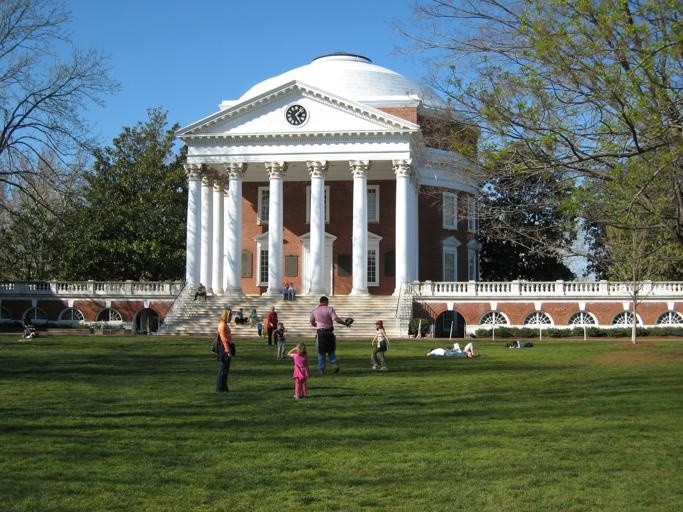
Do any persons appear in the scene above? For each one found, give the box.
[282,283,290,299]
[309,296,353,374]
[211,308,234,390]
[194,283,206,301]
[266,306,278,345]
[272,322,287,357]
[21,323,38,338]
[370,320,389,371]
[248,309,257,327]
[451,342,477,357]
[425,344,467,359]
[235,308,243,325]
[509,339,533,349]
[287,283,295,300]
[256,320,263,337]
[287,343,308,399]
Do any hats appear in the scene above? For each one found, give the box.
[373,320,383,325]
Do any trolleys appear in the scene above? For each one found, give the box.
[25,325,39,339]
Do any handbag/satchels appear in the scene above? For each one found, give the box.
[211,337,235,358]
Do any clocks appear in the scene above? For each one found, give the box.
[284,103,310,128]
